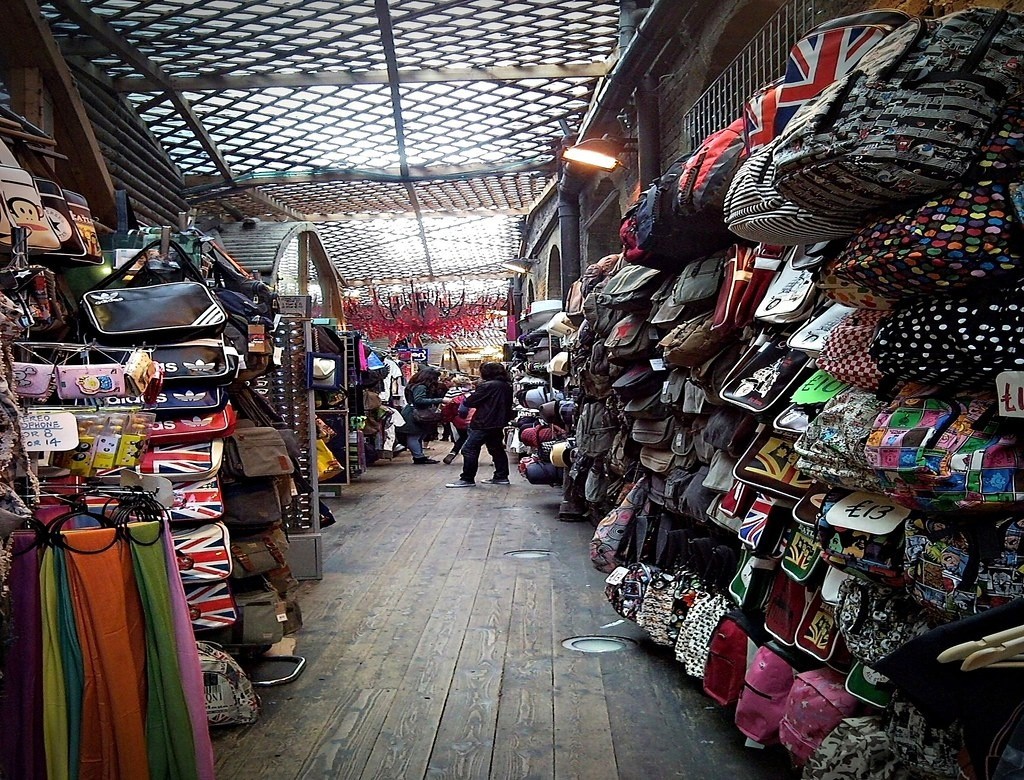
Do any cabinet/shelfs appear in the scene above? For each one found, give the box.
[316,336,350,498]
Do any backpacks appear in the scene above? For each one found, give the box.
[621,7,1024,780]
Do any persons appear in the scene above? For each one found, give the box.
[394,366,454,464]
[441,376,476,464]
[438,374,457,442]
[445,362,513,488]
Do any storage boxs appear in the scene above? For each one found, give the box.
[92,413,128,470]
[61,411,107,478]
[115,412,156,468]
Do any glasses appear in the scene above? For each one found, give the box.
[272,322,312,531]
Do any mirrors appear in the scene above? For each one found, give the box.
[306,351,341,391]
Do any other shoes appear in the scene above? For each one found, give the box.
[414,458,440,464]
[481,478,510,485]
[445,480,476,487]
[443,453,455,464]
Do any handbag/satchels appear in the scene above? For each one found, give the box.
[309,322,343,392]
[359,345,395,463]
[413,405,441,422]
[0,129,298,723]
[316,436,345,482]
[502,9,832,707]
[315,414,338,444]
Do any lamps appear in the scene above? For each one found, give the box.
[500,257,541,274]
[559,135,638,172]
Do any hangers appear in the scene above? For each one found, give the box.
[960,636,1024,672]
[56,333,119,367]
[0,104,57,146]
[936,625,1024,663]
[13,342,53,365]
